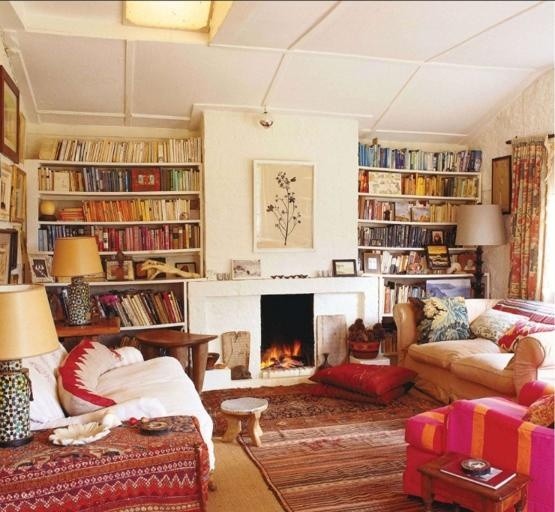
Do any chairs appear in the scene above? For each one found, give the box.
[23,347,217,493]
[402,381,554,512]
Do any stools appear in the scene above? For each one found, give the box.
[223,397,268,447]
[135,330,217,395]
[418,451,531,512]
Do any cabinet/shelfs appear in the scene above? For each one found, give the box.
[359,140,490,356]
[38,136,204,346]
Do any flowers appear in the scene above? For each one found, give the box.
[350,318,385,342]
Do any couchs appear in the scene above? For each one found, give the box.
[393,299,555,405]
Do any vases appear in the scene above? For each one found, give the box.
[350,341,379,360]
[319,352,333,370]
[206,353,219,369]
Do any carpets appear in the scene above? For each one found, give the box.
[201,383,447,437]
[236,415,477,512]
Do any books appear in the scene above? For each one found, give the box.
[0,136,202,327]
[359,138,482,354]
[442,455,516,489]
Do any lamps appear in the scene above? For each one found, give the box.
[455,205,505,299]
[51,235,104,326]
[258,102,273,128]
[1,285,60,446]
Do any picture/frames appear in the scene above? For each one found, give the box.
[332,259,358,277]
[492,155,511,214]
[107,260,134,280]
[363,253,381,274]
[431,229,445,246]
[231,257,263,280]
[1,65,53,283]
[426,244,450,269]
[252,159,316,253]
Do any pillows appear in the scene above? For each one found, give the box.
[523,393,555,428]
[309,364,418,398]
[55,339,123,416]
[470,309,530,343]
[495,320,555,353]
[409,296,476,345]
[312,382,414,405]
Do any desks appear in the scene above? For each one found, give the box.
[55,318,121,351]
[0,416,209,512]
